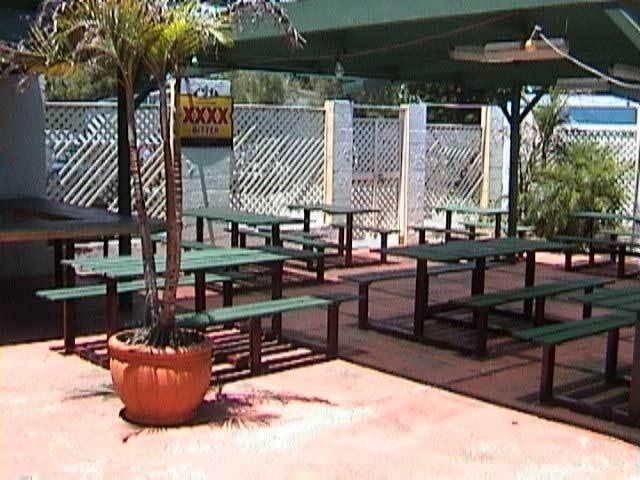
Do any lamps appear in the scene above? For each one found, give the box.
[448,45,513,62]
[334,49,344,78]
[554,76,609,93]
[483,37,568,60]
[609,63,639,88]
[192,51,199,65]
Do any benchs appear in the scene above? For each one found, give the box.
[35,272,256,358]
[151,235,219,250]
[174,293,362,378]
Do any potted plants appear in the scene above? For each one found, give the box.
[0,0,306,426]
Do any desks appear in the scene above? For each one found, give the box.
[1,196,168,311]
[60,246,324,370]
